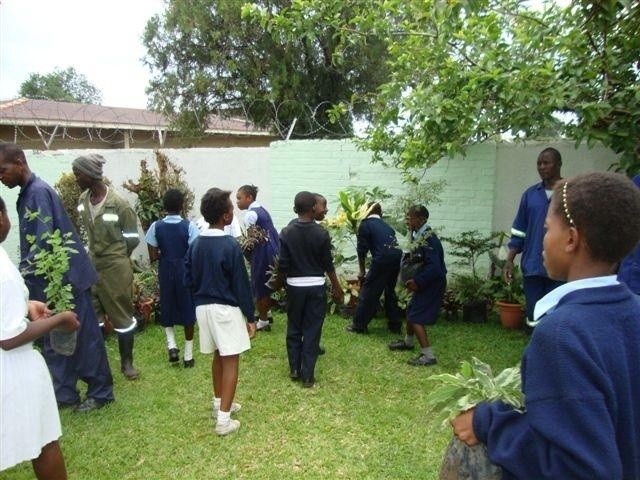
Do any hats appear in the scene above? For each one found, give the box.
[71,154,106,179]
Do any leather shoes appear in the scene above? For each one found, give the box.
[73,395,117,414]
[348,323,369,334]
[389,341,416,351]
[407,354,437,367]
[290,368,314,386]
[183,357,194,368]
[169,348,181,362]
[57,395,82,411]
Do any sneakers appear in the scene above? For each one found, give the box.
[214,419,240,435]
[257,325,271,332]
[212,402,241,415]
[252,314,273,324]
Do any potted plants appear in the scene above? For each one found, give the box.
[489,262,528,328]
[440,229,511,323]
[21,206,80,356]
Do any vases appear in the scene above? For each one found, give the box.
[133,298,154,325]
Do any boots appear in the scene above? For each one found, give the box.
[115,330,139,380]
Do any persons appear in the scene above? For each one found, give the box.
[145,189,201,368]
[184,188,258,436]
[446,171,640,479]
[388,204,447,365]
[235,184,284,333]
[289,192,328,354]
[1,199,83,479]
[346,199,405,335]
[277,190,345,387]
[504,149,565,327]
[0,142,116,412]
[69,153,142,380]
